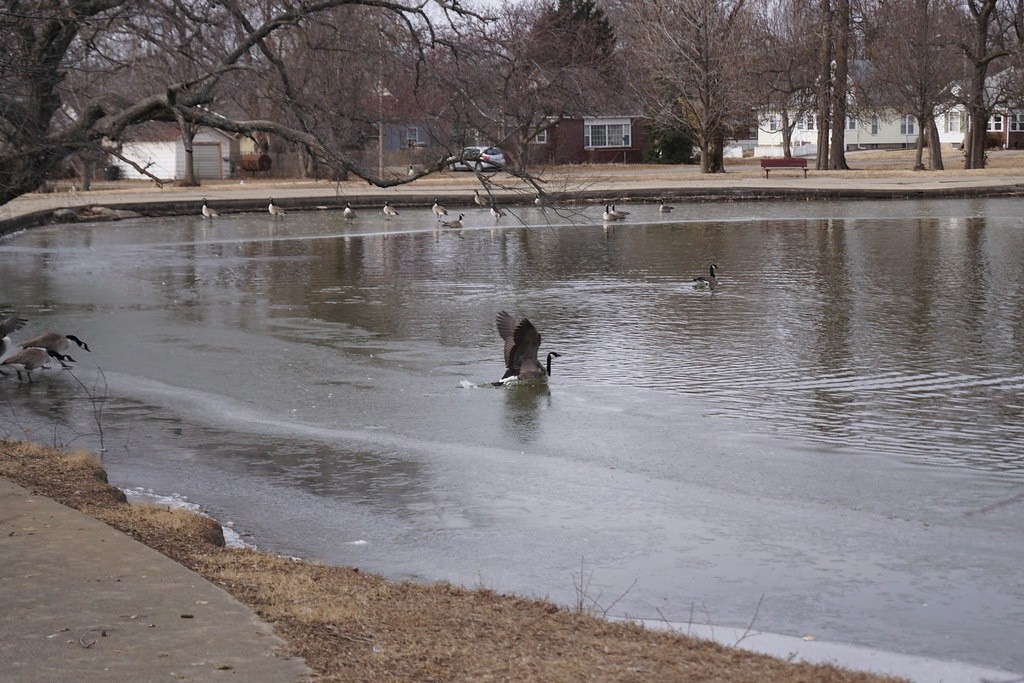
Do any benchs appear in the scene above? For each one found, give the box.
[761,159,809,179]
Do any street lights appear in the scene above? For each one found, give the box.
[934,33,970,156]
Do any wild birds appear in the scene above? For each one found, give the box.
[268,197,288,221]
[693,264,720,290]
[431,198,448,222]
[343,202,358,225]
[0,315,91,383]
[473,190,490,209]
[490,311,563,387]
[659,198,674,212]
[535,195,544,210]
[383,200,399,221]
[603,201,631,221]
[490,202,507,222]
[407,163,417,184]
[441,213,465,228]
[475,156,484,174]
[201,198,223,224]
[448,154,456,172]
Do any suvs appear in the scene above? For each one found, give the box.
[446,146,506,173]
